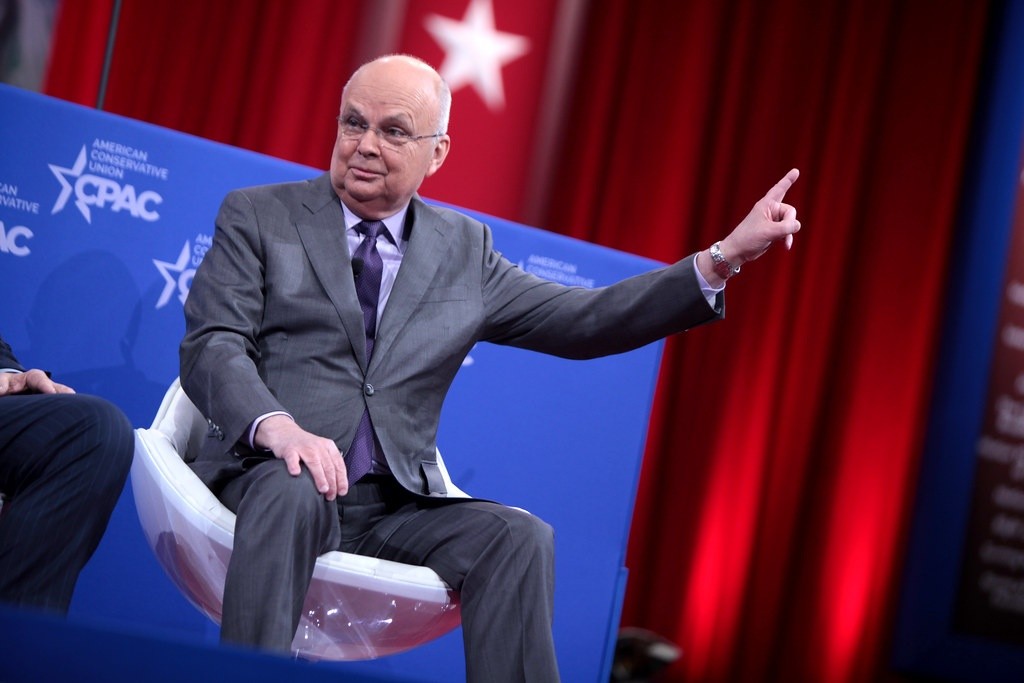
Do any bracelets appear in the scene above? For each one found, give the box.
[710,241,740,281]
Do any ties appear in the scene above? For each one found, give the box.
[343,219,402,487]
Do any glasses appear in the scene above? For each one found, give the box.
[336,113,444,143]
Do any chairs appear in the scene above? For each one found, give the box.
[131,377,532,662]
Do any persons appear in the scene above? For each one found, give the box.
[178,55,801,683]
[0,339,135,617]
